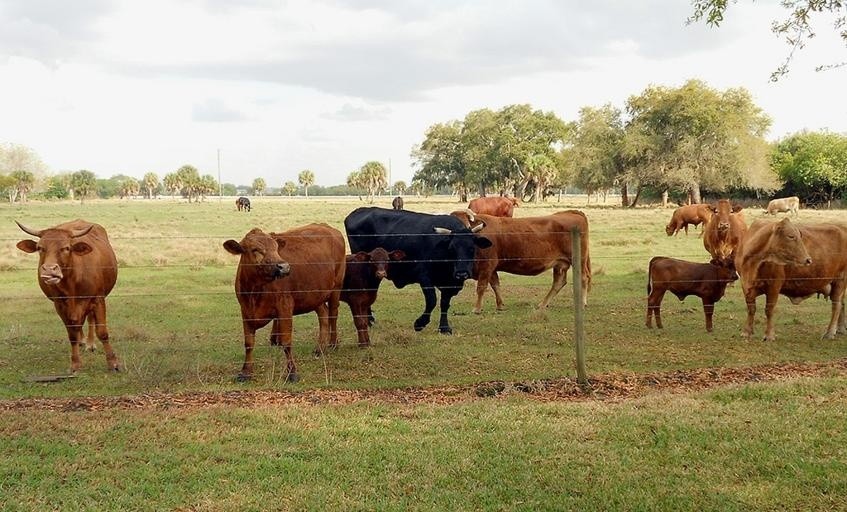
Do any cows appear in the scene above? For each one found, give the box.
[702,197,750,273]
[236,196,250,211]
[665,203,714,239]
[342,205,494,336]
[221,222,345,386]
[391,196,406,208]
[450,209,595,315]
[764,196,799,219]
[268,247,406,348]
[733,214,846,341]
[643,254,740,334]
[468,197,520,220]
[9,215,120,373]
